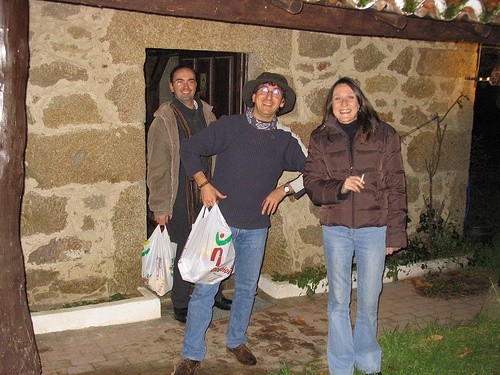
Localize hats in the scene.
[242,72,296,117]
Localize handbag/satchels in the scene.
[141,223,178,296]
[177,201,236,285]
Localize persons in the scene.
[172,72,308,375]
[145,63,234,323]
[304,76,406,375]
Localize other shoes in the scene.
[213,298,233,310]
[174,358,202,375]
[226,342,257,366]
[173,308,187,323]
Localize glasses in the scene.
[172,80,196,86]
[257,87,283,98]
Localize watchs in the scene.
[283,184,290,196]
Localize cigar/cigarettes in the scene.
[360,174,364,183]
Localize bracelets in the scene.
[199,180,209,189]
